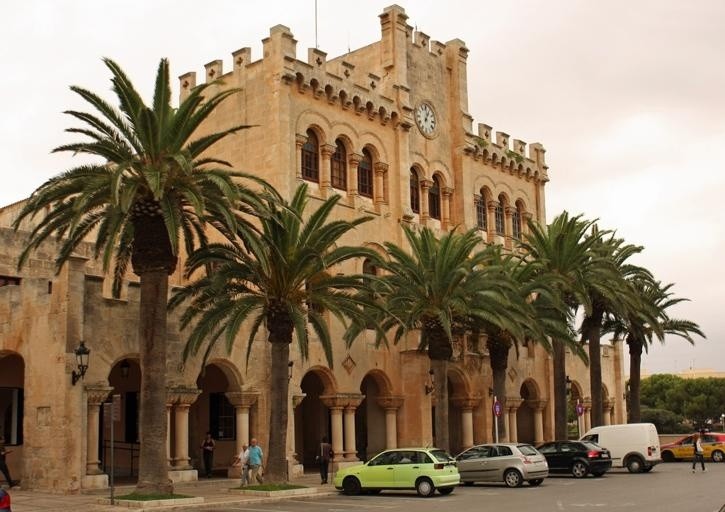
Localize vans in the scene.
[578,423,662,473]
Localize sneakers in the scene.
[691,467,708,473]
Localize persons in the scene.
[0,434,20,488]
[232,443,249,487]
[199,430,216,477]
[0,486,12,511]
[242,438,264,484]
[315,434,334,483]
[692,432,710,473]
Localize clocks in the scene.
[414,99,439,140]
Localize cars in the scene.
[536,439,612,478]
[453,442,549,487]
[660,433,725,463]
[334,447,461,496]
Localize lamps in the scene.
[566,375,572,395]
[71,342,90,386]
[425,369,435,395]
[288,360,293,382]
